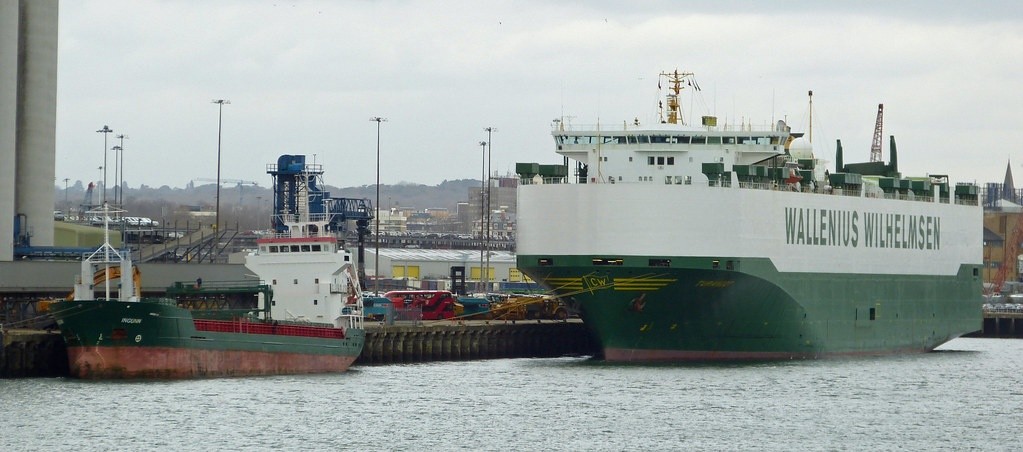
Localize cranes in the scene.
[984,207,1023,298]
[869,103,885,163]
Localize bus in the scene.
[382,289,456,320]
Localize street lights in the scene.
[110,145,123,219]
[95,125,113,212]
[478,140,489,296]
[483,123,500,290]
[369,115,389,297]
[62,178,72,221]
[116,134,130,221]
[211,98,231,268]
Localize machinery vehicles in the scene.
[35,265,142,317]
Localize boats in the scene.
[43,198,368,378]
[514,69,985,362]
